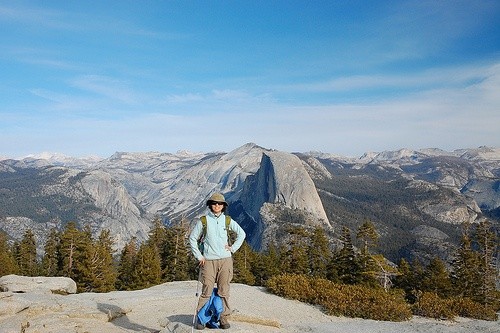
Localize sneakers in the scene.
[197,321,205,330]
[220,316,230,329]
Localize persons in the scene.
[189,192,246,330]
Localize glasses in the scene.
[211,201,223,205]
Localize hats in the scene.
[206,193,229,206]
